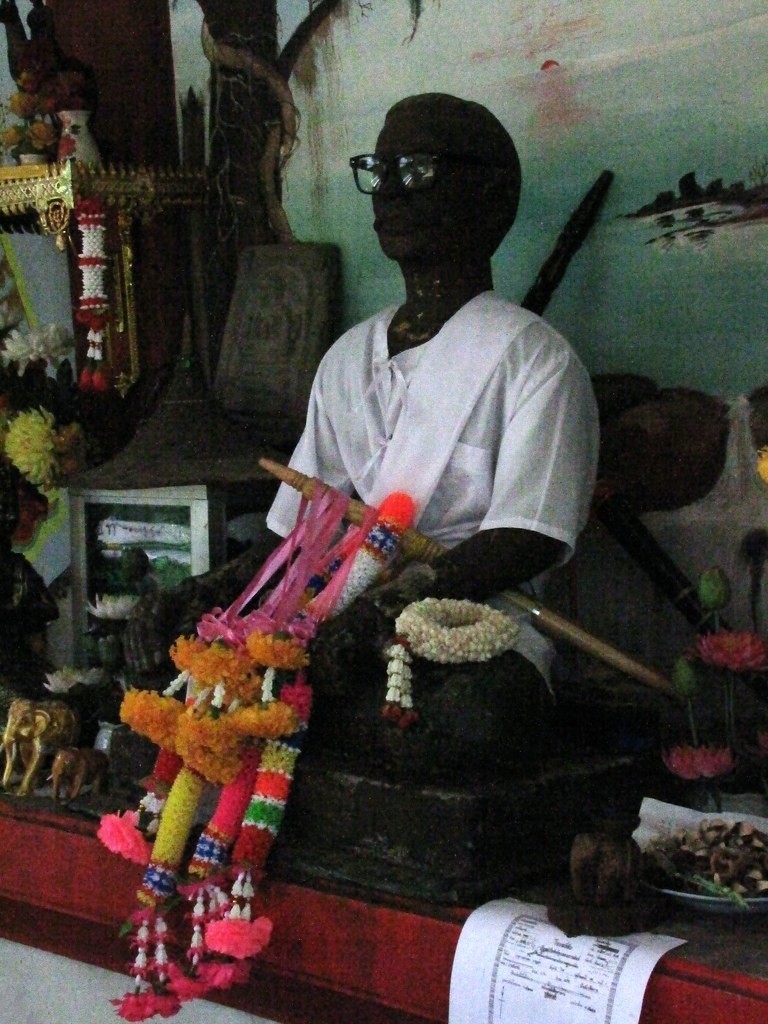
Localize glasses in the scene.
[350,148,504,194]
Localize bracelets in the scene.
[377,598,523,729]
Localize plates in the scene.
[643,881,767,913]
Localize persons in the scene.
[117,92,602,685]
